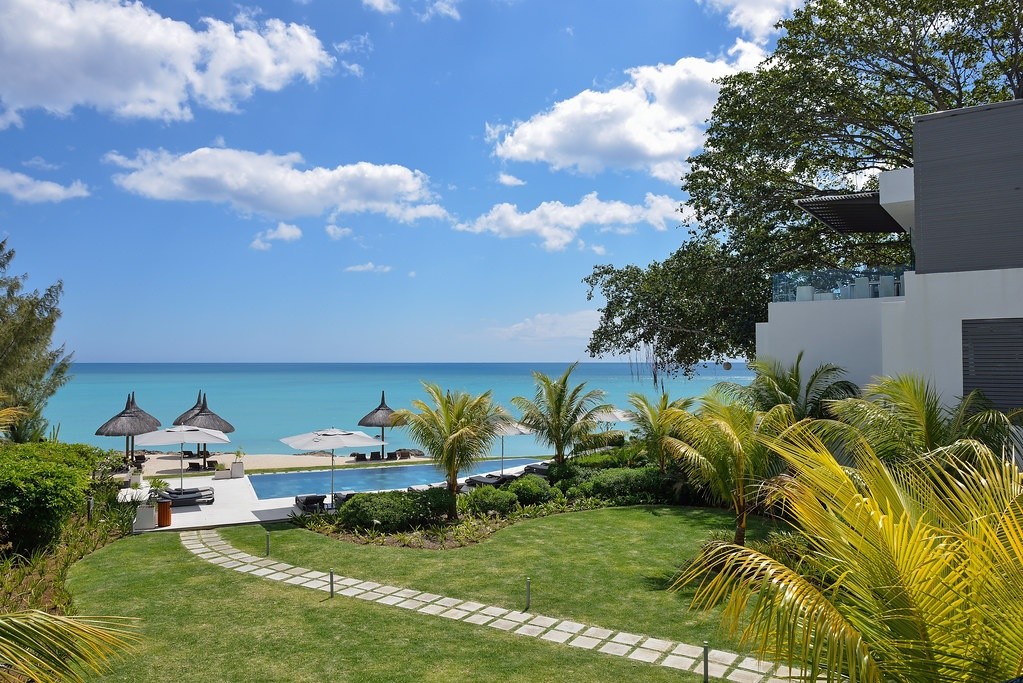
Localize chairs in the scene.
[132,462,142,471]
[186,462,204,471]
[370,451,382,461]
[180,450,216,458]
[295,494,328,515]
[524,462,549,474]
[386,451,411,460]
[334,493,362,506]
[134,455,150,463]
[408,482,463,494]
[464,472,535,488]
[356,452,367,461]
[149,487,215,507]
[207,460,224,471]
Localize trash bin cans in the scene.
[135,505,157,530]
[158,499,172,527]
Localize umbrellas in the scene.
[596,408,632,429]
[93,389,234,472]
[135,423,231,496]
[358,390,408,459]
[279,425,390,509]
[494,420,538,476]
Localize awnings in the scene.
[793,192,906,236]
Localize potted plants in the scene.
[147,475,170,504]
[213,462,232,478]
[230,447,247,478]
[129,469,143,489]
[136,496,157,529]
